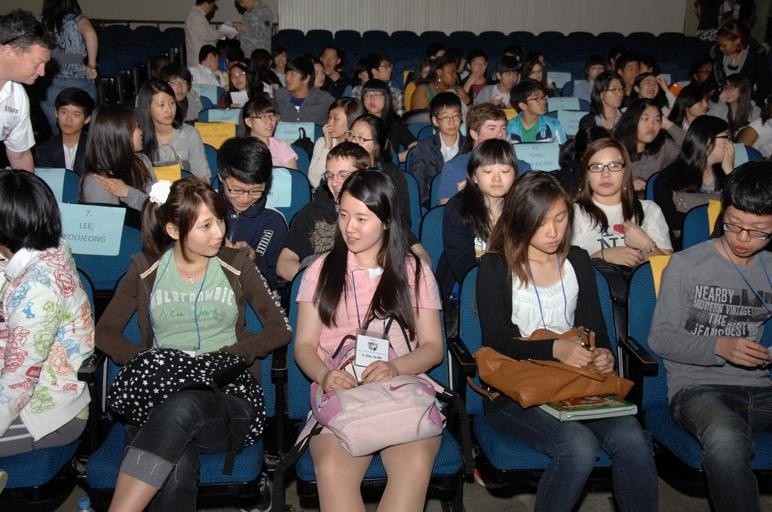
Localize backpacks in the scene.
[311,335,446,456]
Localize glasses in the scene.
[321,171,351,180]
[2,21,46,46]
[715,135,732,140]
[722,221,771,240]
[224,178,265,195]
[251,115,277,121]
[587,162,626,172]
[604,88,625,94]
[344,131,375,144]
[363,94,385,100]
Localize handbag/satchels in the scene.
[291,127,315,160]
[105,347,267,449]
[474,329,634,408]
[673,191,722,213]
[591,258,634,336]
[151,144,191,182]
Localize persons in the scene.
[35,45,771,487]
[92,178,290,512]
[2,1,97,173]
[476,171,657,511]
[184,1,273,68]
[648,157,771,511]
[293,170,446,512]
[1,169,94,496]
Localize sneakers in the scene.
[474,464,505,488]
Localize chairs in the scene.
[0,25,772,512]
